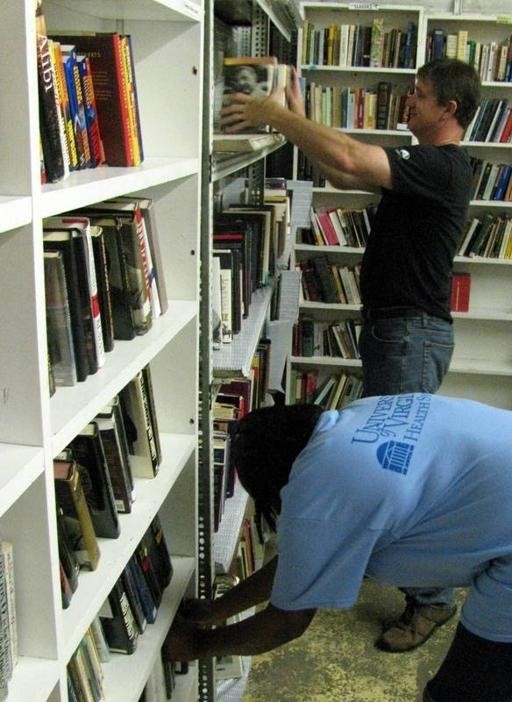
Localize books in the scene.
[0,666,9,700]
[0,553,13,680]
[2,539,20,667]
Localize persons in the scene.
[162,390,510,698]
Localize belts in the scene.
[359,306,436,321]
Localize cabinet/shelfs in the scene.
[420,13,512,413]
[283,1,428,405]
[0,0,209,702]
[206,1,307,702]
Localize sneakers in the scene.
[372,594,459,654]
